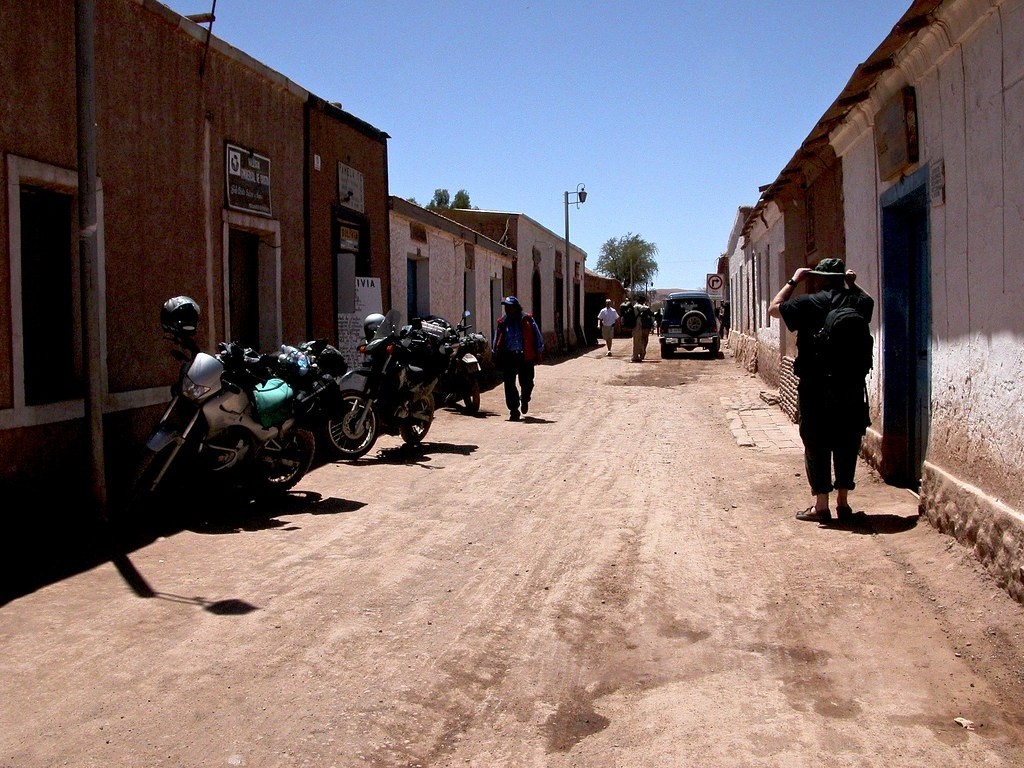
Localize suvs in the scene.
[657,292,721,359]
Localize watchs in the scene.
[786,278,797,288]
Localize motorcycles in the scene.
[323,307,461,460]
[113,293,347,522]
[438,308,489,414]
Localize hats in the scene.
[501,296,519,307]
[807,258,845,276]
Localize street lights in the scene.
[564,183,588,354]
[645,281,654,299]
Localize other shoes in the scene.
[641,355,644,359]
[795,505,832,522]
[606,351,611,356]
[632,358,641,363]
[509,410,520,420]
[836,504,853,520]
[521,398,528,414]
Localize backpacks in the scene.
[807,290,873,379]
[619,301,643,329]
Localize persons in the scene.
[654,309,663,337]
[768,258,875,522]
[631,296,655,361]
[719,296,730,340]
[597,299,621,356]
[491,295,543,420]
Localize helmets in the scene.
[363,313,394,344]
[160,295,201,337]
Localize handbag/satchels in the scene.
[298,340,348,378]
[449,331,487,371]
[294,376,341,431]
[218,340,272,381]
[400,314,454,366]
